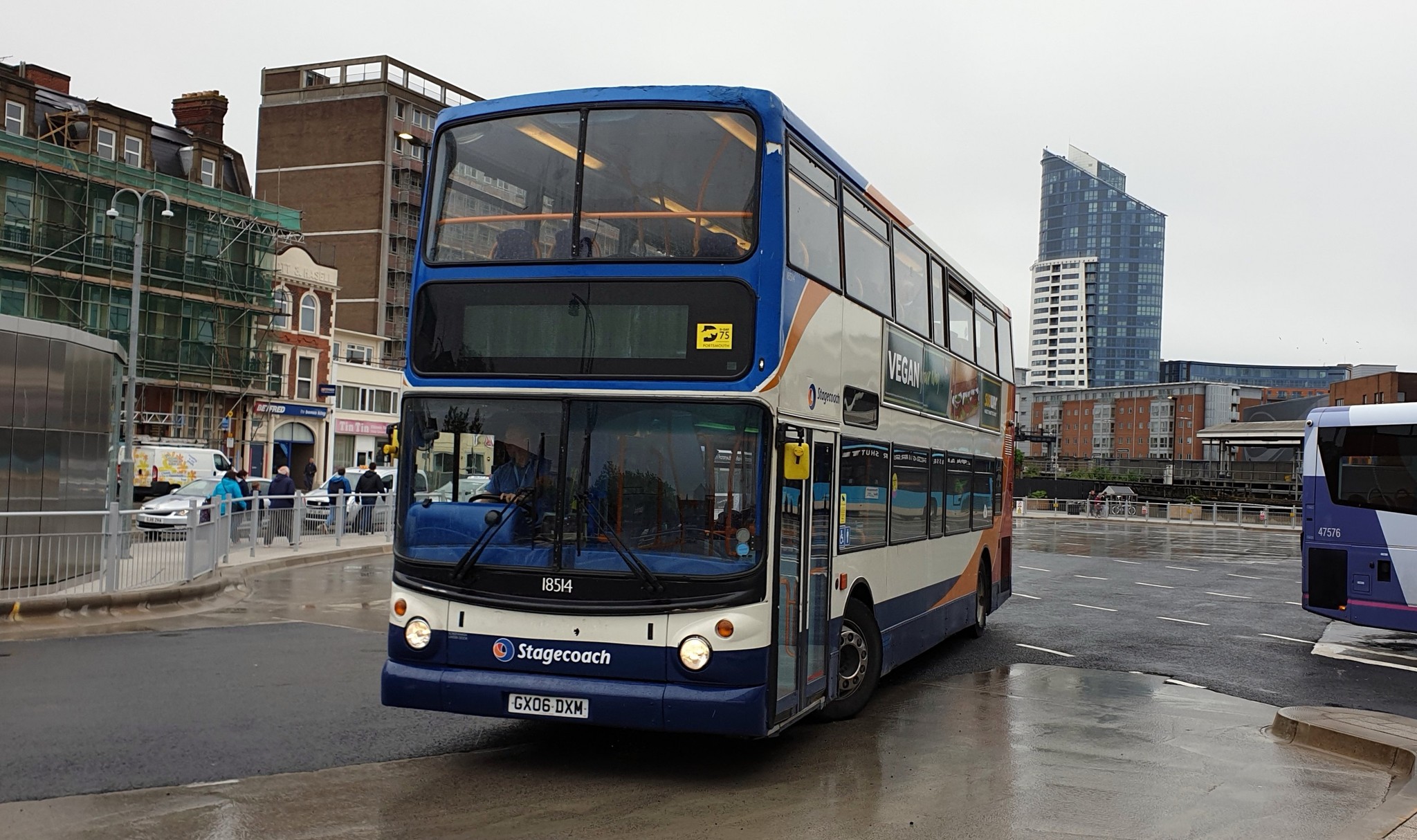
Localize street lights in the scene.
[1166,396,1179,485]
[106,187,175,562]
[567,292,598,374]
[1048,428,1058,512]
[1102,419,1115,459]
[1177,417,1192,476]
[1014,410,1018,480]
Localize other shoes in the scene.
[321,524,328,533]
[289,541,302,545]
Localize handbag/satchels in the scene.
[231,502,238,513]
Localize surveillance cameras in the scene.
[106,210,119,220]
[161,210,174,219]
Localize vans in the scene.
[106,434,242,502]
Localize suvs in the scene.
[303,466,429,532]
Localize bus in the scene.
[780,443,992,522]
[382,80,1018,742]
[1300,402,1417,635]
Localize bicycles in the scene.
[1111,495,1136,515]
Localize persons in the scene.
[302,458,316,492]
[1088,490,1107,518]
[355,462,386,536]
[323,468,351,534]
[595,437,679,545]
[480,425,553,511]
[212,469,265,547]
[264,466,295,548]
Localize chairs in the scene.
[1350,488,1414,508]
[488,228,970,368]
[713,505,961,544]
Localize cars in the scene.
[134,474,309,540]
[430,476,492,502]
